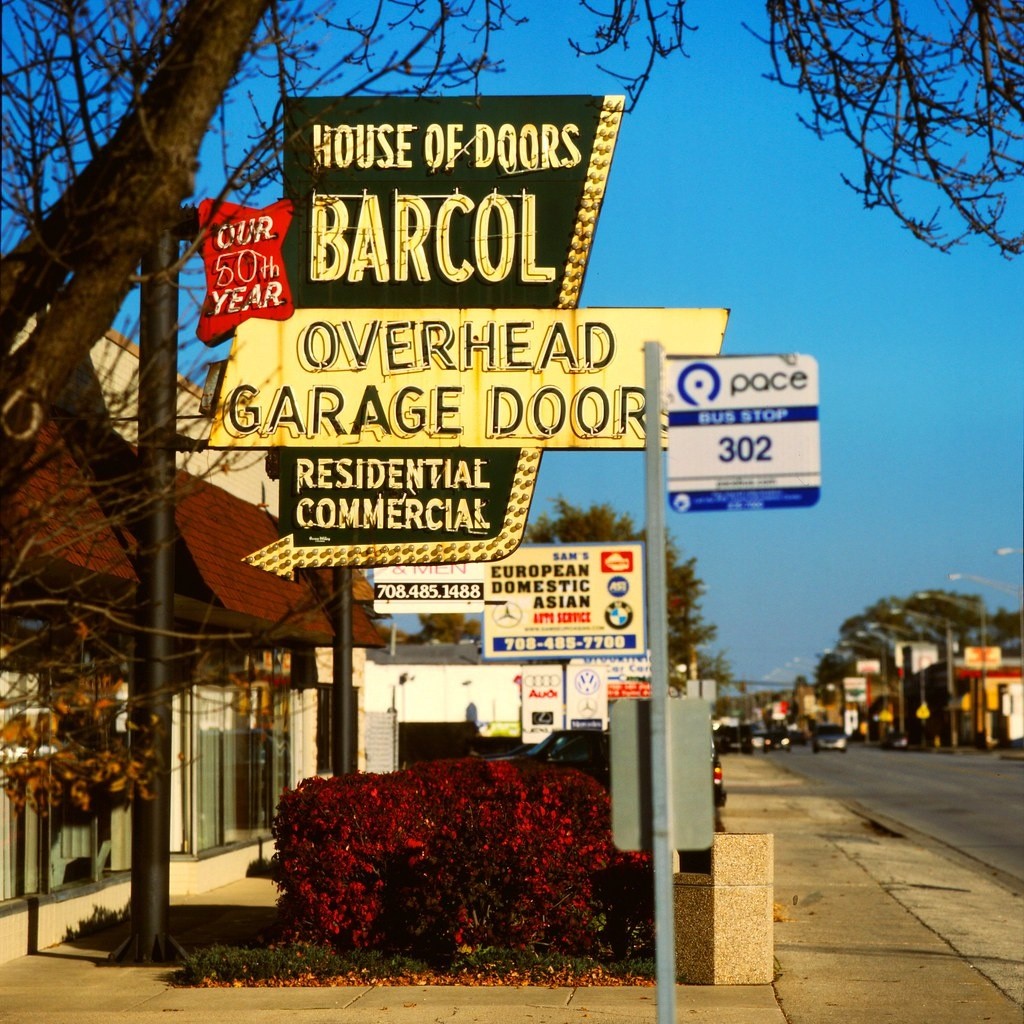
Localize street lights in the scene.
[948,574,1024,675]
[888,608,957,748]
[916,592,991,752]
[866,622,930,750]
[824,631,900,747]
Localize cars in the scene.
[811,724,848,754]
[713,719,806,754]
